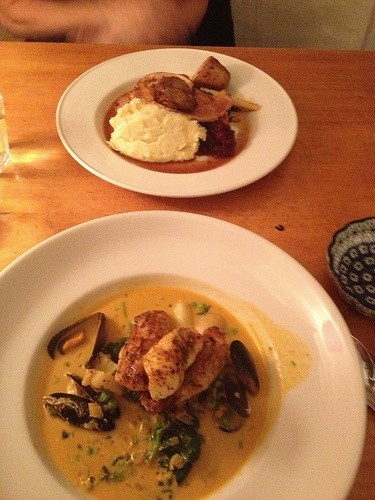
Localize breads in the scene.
[109,97,207,162]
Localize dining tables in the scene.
[0,41,375,500]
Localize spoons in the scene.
[352,335,375,411]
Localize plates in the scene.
[1,210,366,499]
[56,48,299,199]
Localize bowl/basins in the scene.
[328,217,375,317]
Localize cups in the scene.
[0,94,12,171]
